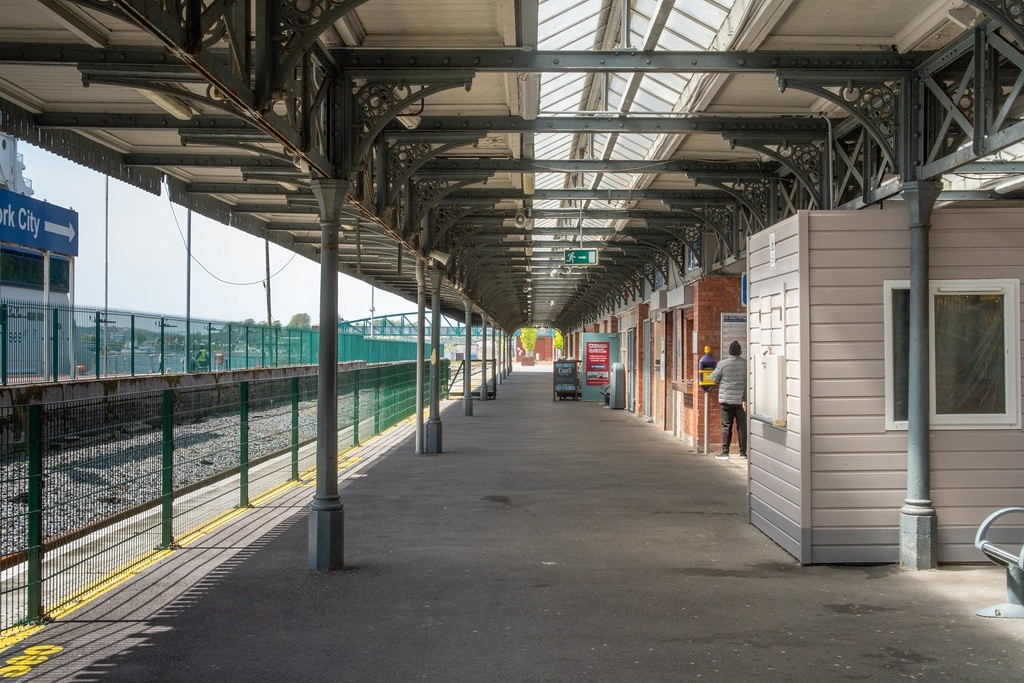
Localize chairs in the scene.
[974,507,1024,618]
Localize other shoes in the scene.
[737,453,747,459]
[715,453,729,460]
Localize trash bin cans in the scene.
[553,359,578,401]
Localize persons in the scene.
[158,351,166,372]
[214,349,224,371]
[181,353,185,372]
[195,342,209,372]
[708,340,747,460]
[255,358,262,366]
